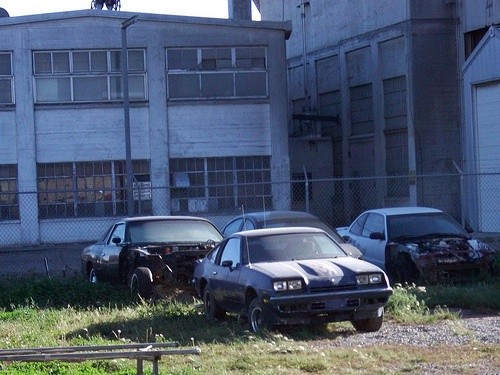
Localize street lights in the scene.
[119,15,138,218]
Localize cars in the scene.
[333,207,496,285]
[80,216,226,308]
[193,227,394,339]
[218,210,363,259]
